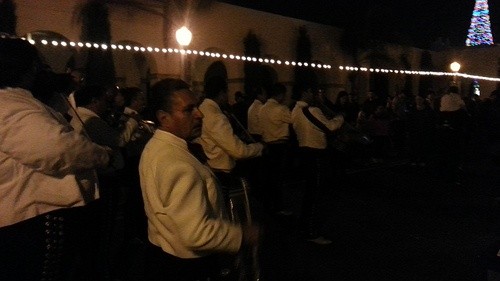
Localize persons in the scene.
[0,36,118,281]
[138,77,265,281]
[47,55,499,246]
